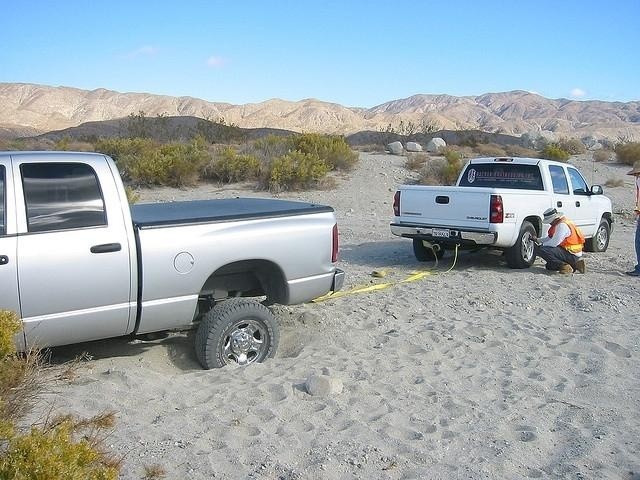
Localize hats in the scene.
[626,160,640,175]
[541,207,563,224]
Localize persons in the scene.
[625,159,640,276]
[529,207,586,275]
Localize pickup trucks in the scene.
[0,151,345,370]
[390,156,616,269]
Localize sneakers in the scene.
[558,262,574,275]
[574,258,586,274]
[626,268,640,276]
